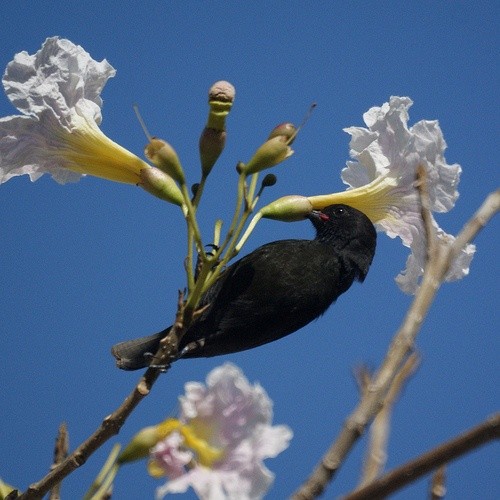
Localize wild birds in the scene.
[111,203,378,371]
